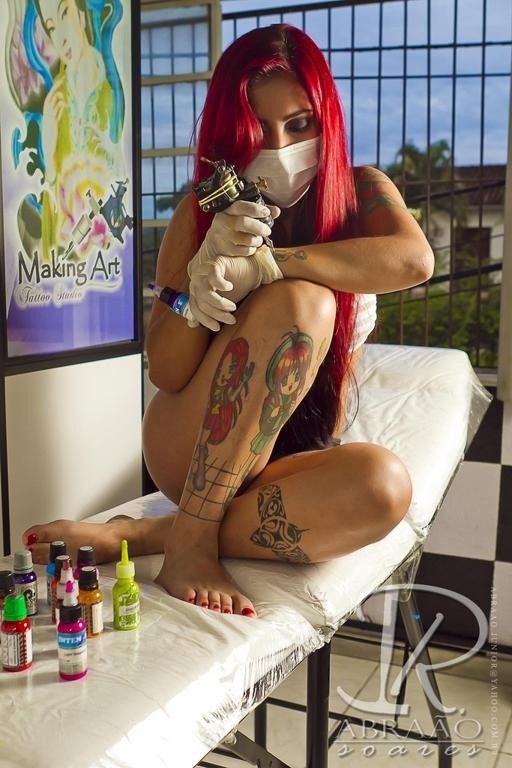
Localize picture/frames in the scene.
[0,0,145,378]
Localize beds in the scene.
[0,344,474,767]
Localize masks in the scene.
[241,134,320,209]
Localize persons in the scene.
[32,1,117,269]
[22,20,435,622]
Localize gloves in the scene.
[186,245,284,332]
[187,201,282,279]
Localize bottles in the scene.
[111,538,140,629]
[1,541,104,680]
[143,280,197,318]
